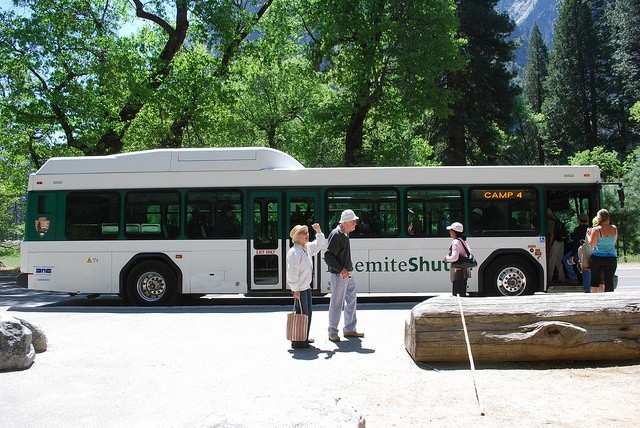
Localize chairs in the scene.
[162,224,170,238]
[126,223,141,234]
[200,224,208,238]
[140,223,162,235]
[101,222,119,235]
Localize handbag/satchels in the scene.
[448,253,477,268]
[286,313,308,341]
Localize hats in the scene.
[433,208,443,212]
[360,208,369,213]
[579,214,588,220]
[410,208,418,213]
[290,225,308,244]
[472,208,483,216]
[446,222,464,232]
[339,209,359,223]
[296,205,303,209]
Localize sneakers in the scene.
[306,339,314,343]
[566,279,578,283]
[344,332,364,337]
[292,341,309,348]
[329,334,340,341]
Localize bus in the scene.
[19,147,624,307]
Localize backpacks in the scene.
[547,218,569,242]
[576,231,593,273]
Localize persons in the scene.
[284,222,326,349]
[558,213,591,287]
[209,203,240,238]
[289,200,313,247]
[188,209,204,238]
[546,216,568,286]
[586,209,618,292]
[546,205,579,238]
[432,204,452,237]
[407,206,423,235]
[321,208,366,341]
[471,207,488,229]
[442,221,471,296]
[578,215,598,293]
